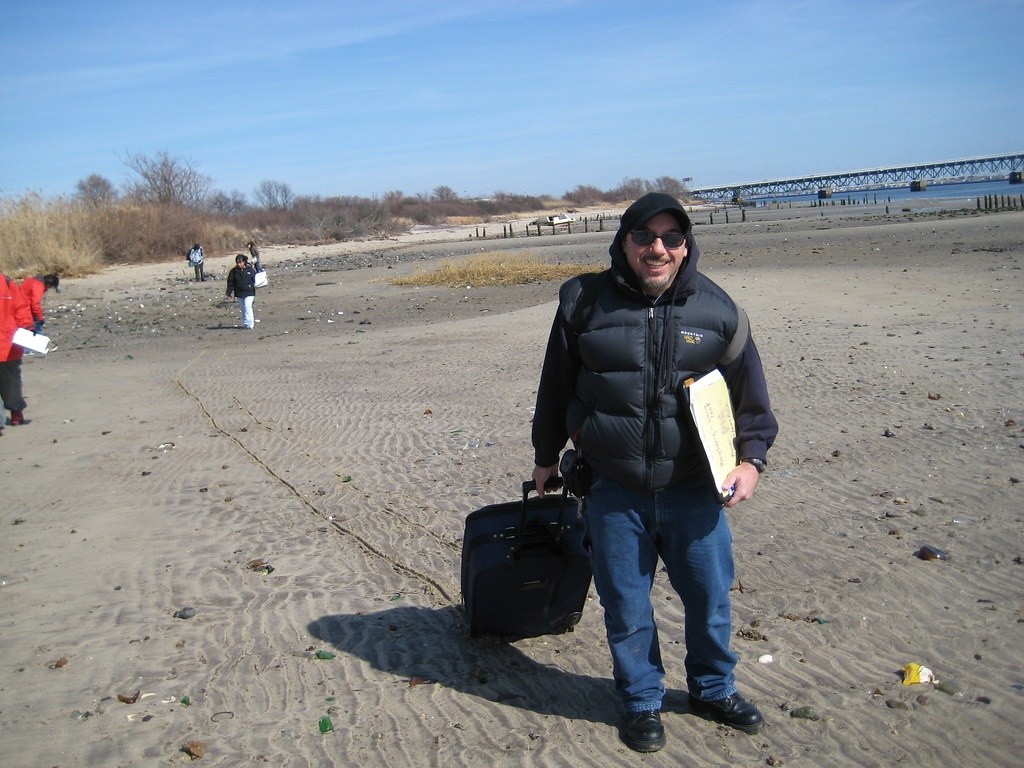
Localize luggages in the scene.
[458,478,592,647]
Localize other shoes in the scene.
[10,411,23,424]
[23,351,34,357]
[49,346,58,352]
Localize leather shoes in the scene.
[624,709,665,752]
[688,692,765,733]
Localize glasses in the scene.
[627,230,687,249]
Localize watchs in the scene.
[740,457,765,473]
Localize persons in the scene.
[246,241,264,273]
[0,271,38,426]
[531,192,780,753]
[189,243,207,282]
[0,395,5,433]
[19,272,62,356]
[226,254,268,329]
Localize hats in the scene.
[620,193,689,246]
